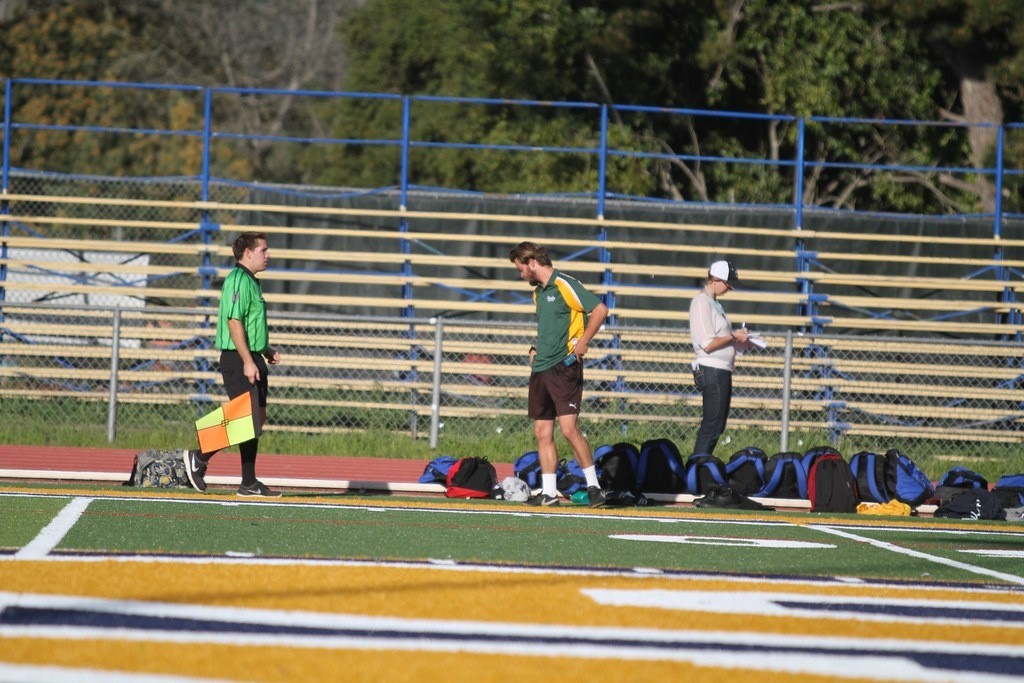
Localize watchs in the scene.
[528,345,537,353]
[730,334,738,343]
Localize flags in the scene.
[194,388,262,456]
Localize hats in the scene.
[710,261,743,292]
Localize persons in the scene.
[689,260,755,463]
[182,230,282,499]
[508,242,608,506]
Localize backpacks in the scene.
[445,456,497,499]
[418,457,459,484]
[514,438,1024,513]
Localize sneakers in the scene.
[522,491,560,507]
[587,486,607,508]
[237,480,282,497]
[182,449,207,493]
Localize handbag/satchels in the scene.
[934,492,1007,519]
[122,450,193,488]
[495,477,530,502]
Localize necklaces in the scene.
[703,284,725,314]
